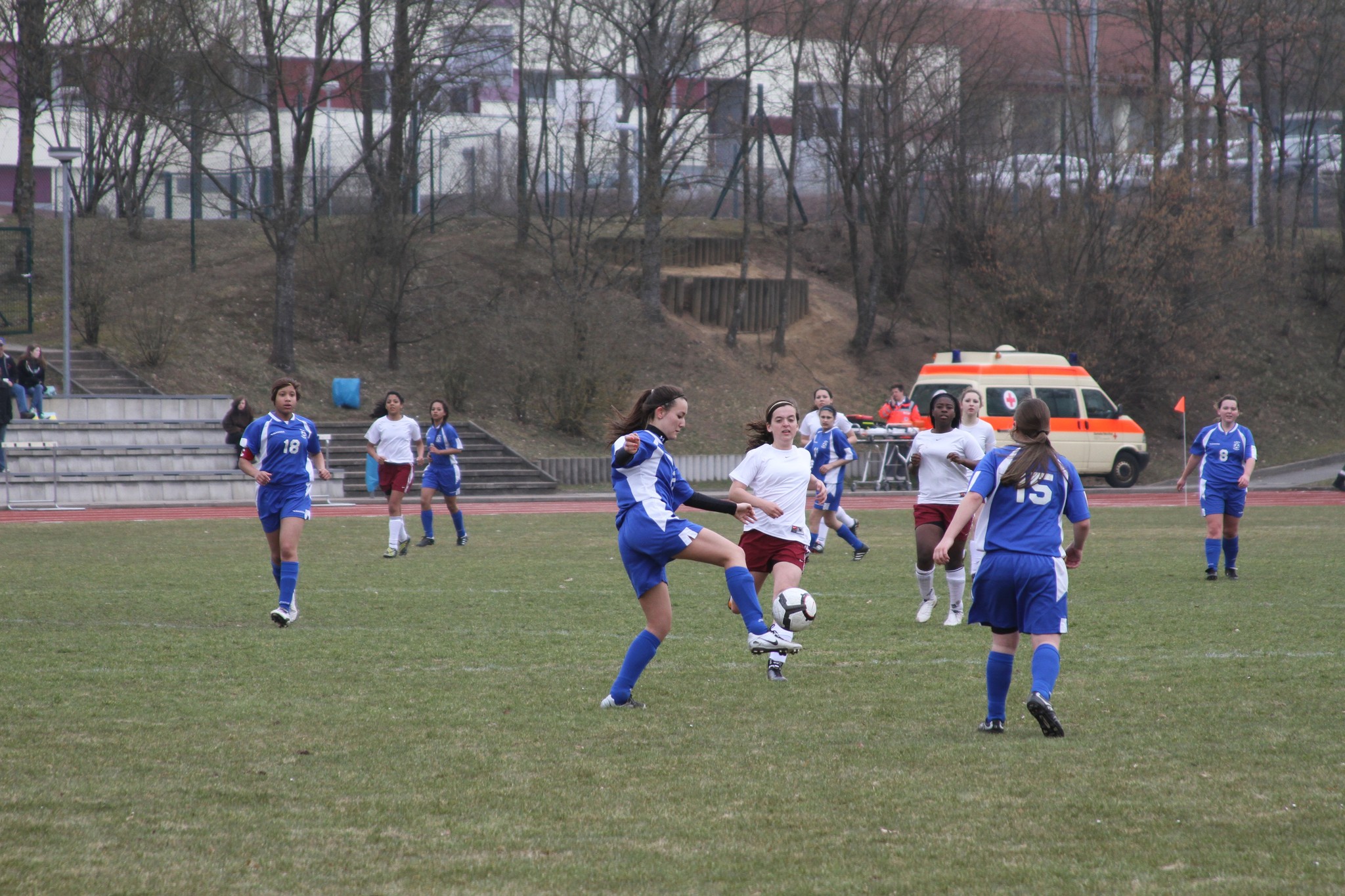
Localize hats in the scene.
[0,338,5,344]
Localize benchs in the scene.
[0,391,350,506]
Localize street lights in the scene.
[322,80,339,211]
[48,146,83,397]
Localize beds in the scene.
[849,423,921,488]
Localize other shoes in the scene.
[1,468,11,472]
[31,407,50,419]
[20,412,35,419]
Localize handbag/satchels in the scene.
[44,385,57,397]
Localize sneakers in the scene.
[416,536,435,547]
[851,543,870,562]
[747,630,803,656]
[1225,567,1239,579]
[917,595,937,623]
[399,538,410,556]
[456,532,468,546]
[811,541,824,553]
[270,609,290,629]
[766,668,789,684]
[383,547,398,558]
[944,610,964,626]
[1026,691,1065,739]
[288,589,298,623]
[848,518,860,540]
[1205,567,1218,580]
[978,717,1005,733]
[600,694,646,710]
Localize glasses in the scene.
[0,343,4,347]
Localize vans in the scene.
[909,344,1148,488]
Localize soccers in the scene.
[772,588,816,634]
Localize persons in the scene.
[934,396,1091,740]
[597,384,802,710]
[907,393,987,628]
[222,396,259,469]
[954,387,997,584]
[1175,394,1258,580]
[726,400,828,680]
[0,337,36,420]
[798,386,860,547]
[802,404,870,561]
[879,384,922,491]
[239,376,332,624]
[416,399,469,548]
[0,368,13,472]
[364,391,425,557]
[16,343,50,419]
